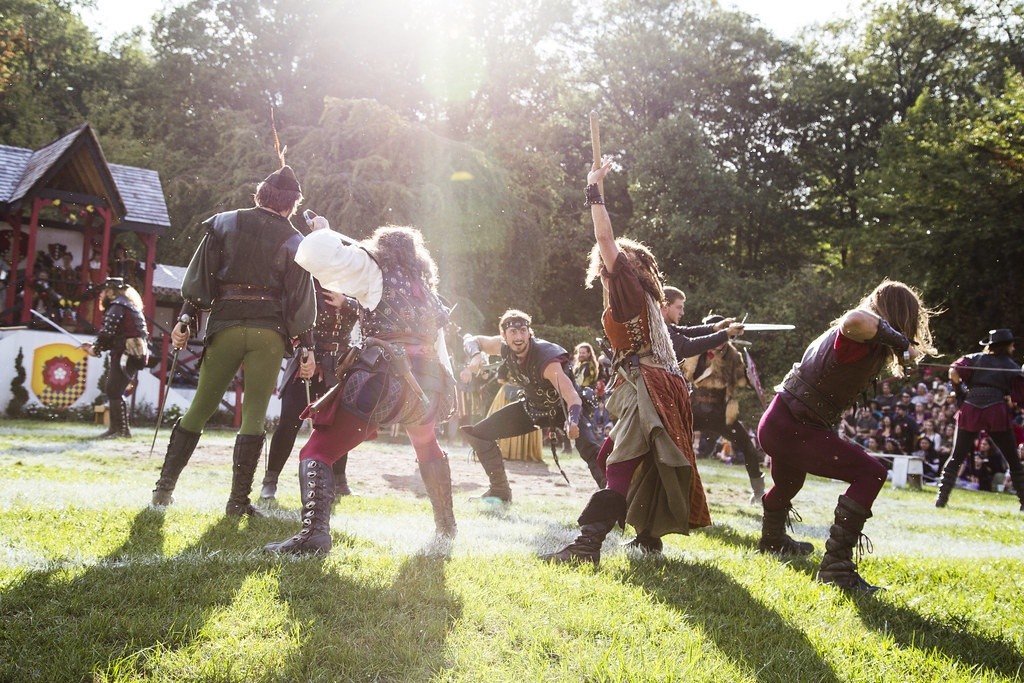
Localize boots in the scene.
[333,474,351,496]
[105,400,134,437]
[260,470,279,502]
[415,451,459,556]
[458,425,514,503]
[750,473,766,505]
[225,430,267,516]
[1009,469,1024,510]
[816,494,887,597]
[578,440,608,490]
[536,490,629,575]
[934,458,963,507]
[759,493,815,558]
[151,418,204,506]
[102,401,117,437]
[618,529,664,554]
[262,458,335,553]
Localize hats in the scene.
[96,277,129,290]
[263,165,302,193]
[979,329,1023,345]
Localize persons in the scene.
[443,286,768,505]
[839,362,1024,495]
[0,239,149,439]
[534,161,712,572]
[152,166,457,557]
[459,308,627,530]
[936,328,1024,511]
[755,278,937,594]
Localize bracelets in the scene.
[585,185,605,205]
